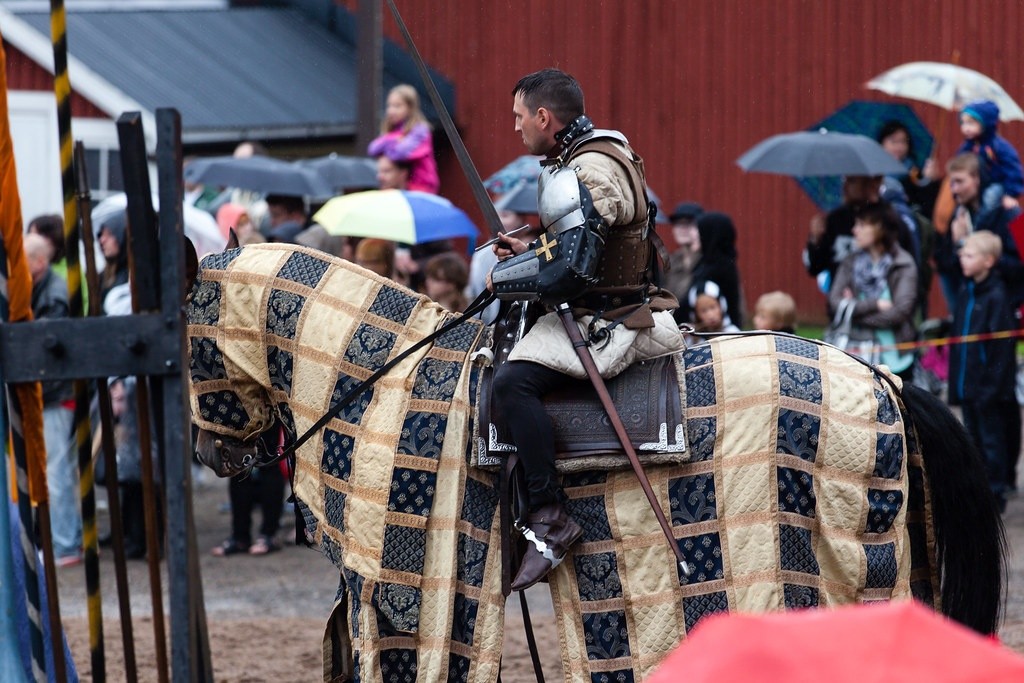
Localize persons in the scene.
[664,203,743,337]
[21,209,168,564]
[462,206,546,325]
[485,67,679,592]
[800,101,1024,517]
[178,142,469,556]
[368,84,440,193]
[752,290,797,334]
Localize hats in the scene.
[669,202,704,224]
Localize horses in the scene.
[184,224,1008,683]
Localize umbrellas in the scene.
[82,193,224,259]
[793,101,935,216]
[311,189,481,244]
[301,155,382,187]
[734,127,909,177]
[865,60,1024,124]
[181,155,325,196]
[482,155,671,223]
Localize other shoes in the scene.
[35,548,79,567]
[211,537,250,556]
[118,543,147,557]
[248,538,279,553]
[97,529,120,547]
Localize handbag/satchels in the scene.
[824,299,880,366]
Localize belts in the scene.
[569,284,649,311]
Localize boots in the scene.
[510,504,583,592]
